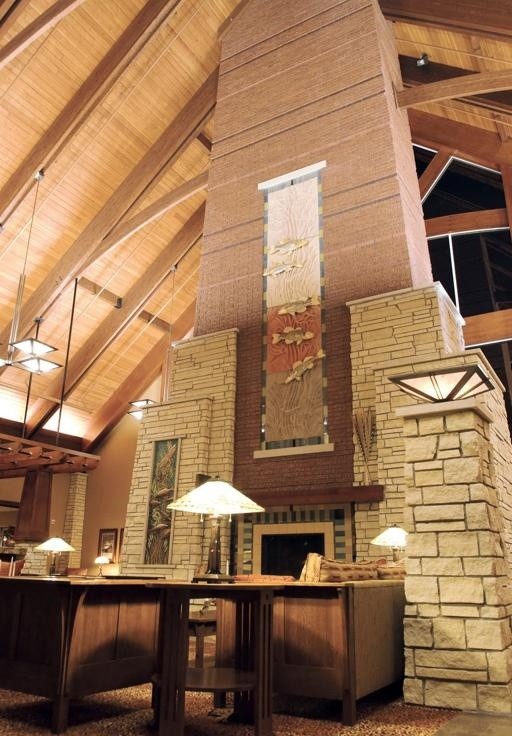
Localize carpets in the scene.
[0,639,462,736]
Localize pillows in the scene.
[298,552,405,583]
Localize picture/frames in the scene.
[97,527,126,564]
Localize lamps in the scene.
[386,363,494,403]
[165,478,265,585]
[6,315,64,375]
